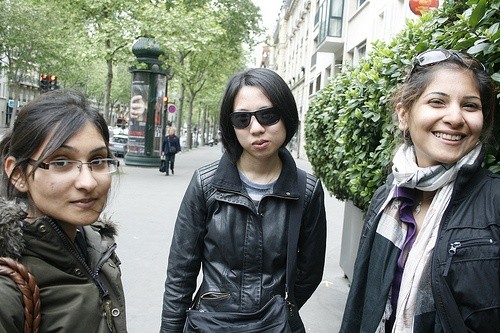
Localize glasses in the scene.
[28,150,119,177]
[228,105,281,129]
[411,48,486,73]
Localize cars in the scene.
[109,134,129,157]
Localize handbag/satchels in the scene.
[182,292,308,333]
[159,154,167,172]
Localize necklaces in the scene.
[414,197,435,215]
[239,160,280,185]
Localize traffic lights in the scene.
[40,74,49,94]
[49,75,57,91]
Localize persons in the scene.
[338,49,500,333]
[129,87,147,154]
[160,68,327,333]
[161,128,181,176]
[0,89,126,333]
